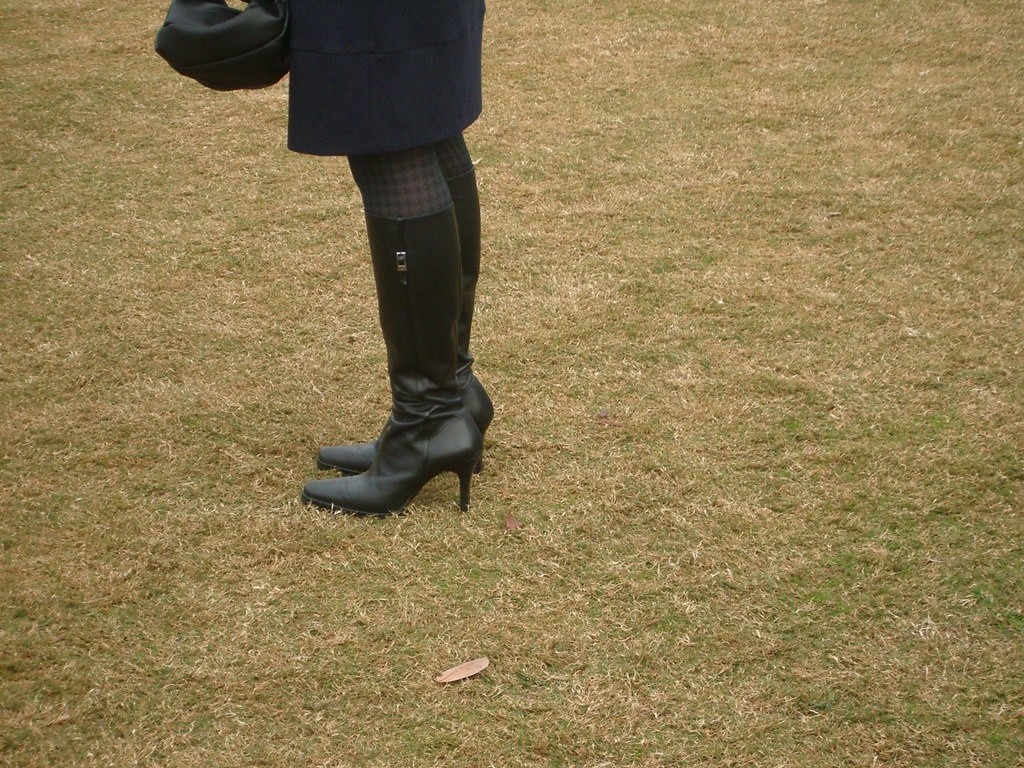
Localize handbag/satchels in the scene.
[155,0,290,91]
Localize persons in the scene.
[275,0,493,517]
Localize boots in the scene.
[299,160,496,515]
